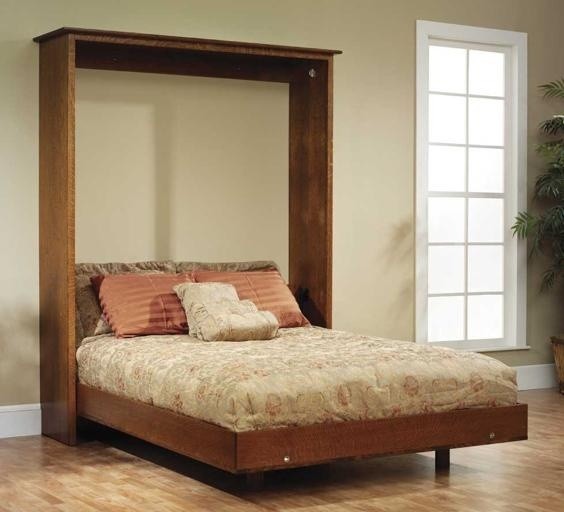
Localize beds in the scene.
[72,262,528,493]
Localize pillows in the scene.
[173,282,239,337]
[191,268,308,328]
[200,310,277,342]
[193,299,258,330]
[89,272,196,338]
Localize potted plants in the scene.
[510,78,564,393]
